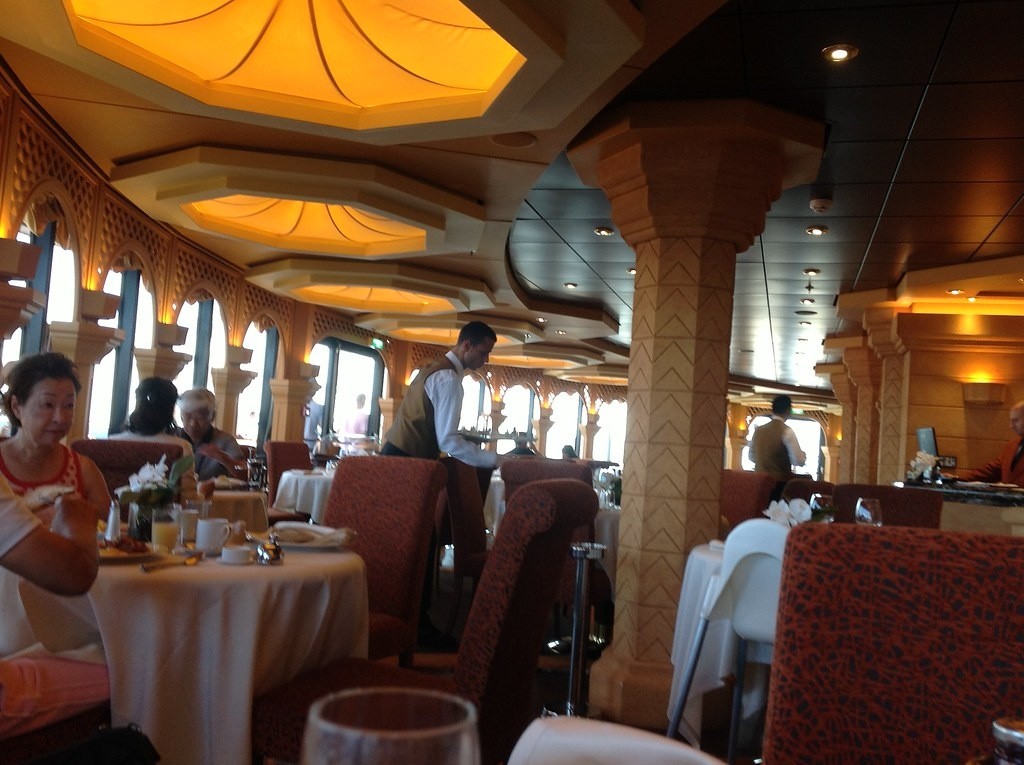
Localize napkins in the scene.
[708,538,726,553]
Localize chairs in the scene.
[1,438,1024,764]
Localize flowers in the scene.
[117,453,196,507]
[760,497,836,530]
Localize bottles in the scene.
[991,716,1024,765]
[613,469,622,506]
[104,499,122,548]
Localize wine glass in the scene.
[592,468,616,512]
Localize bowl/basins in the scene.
[222,546,250,562]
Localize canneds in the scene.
[990,715,1024,765]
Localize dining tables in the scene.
[273,469,336,524]
[664,540,778,765]
[590,504,621,599]
[482,477,507,535]
[81,526,367,765]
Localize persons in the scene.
[303,393,369,451]
[0,470,98,657]
[749,395,806,473]
[176,388,248,483]
[109,376,199,480]
[380,321,502,468]
[503,432,544,457]
[0,352,112,529]
[1008,401,1024,489]
[562,445,580,459]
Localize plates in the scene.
[97,520,341,564]
[215,478,244,488]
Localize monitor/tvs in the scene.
[918,428,939,484]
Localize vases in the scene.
[127,498,171,539]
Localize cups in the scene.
[296,686,483,765]
[185,499,213,519]
[195,518,231,551]
[809,493,834,523]
[854,497,882,526]
[151,503,183,553]
[182,509,199,541]
[328,459,341,474]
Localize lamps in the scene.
[0,0,1024,410]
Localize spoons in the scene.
[140,551,205,571]
